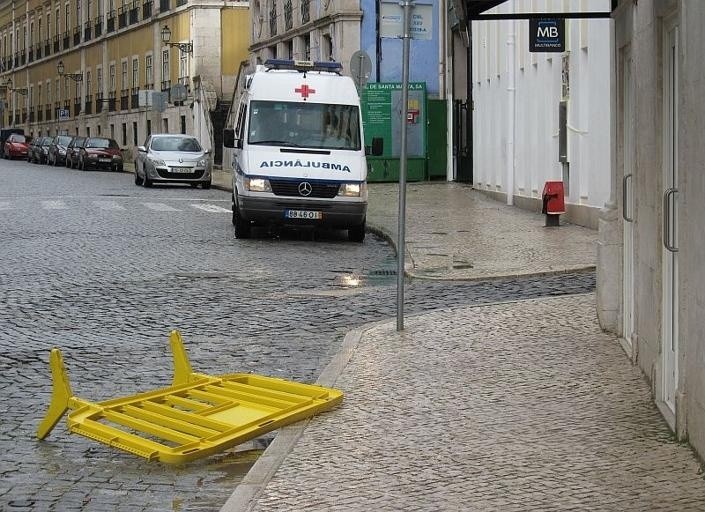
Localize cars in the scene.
[133,130,217,192]
[2,131,125,173]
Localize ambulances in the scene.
[222,56,373,244]
